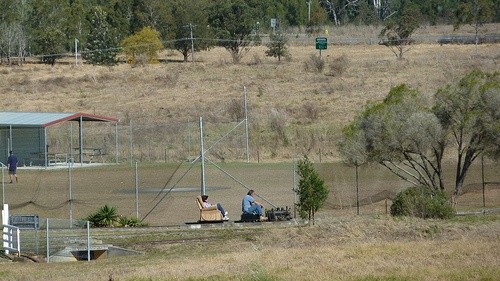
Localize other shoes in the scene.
[260,216,268,221]
[224,211,228,216]
[223,216,229,220]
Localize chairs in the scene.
[197,196,224,221]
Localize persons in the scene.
[242,190,268,221]
[7,150,18,183]
[202,195,230,220]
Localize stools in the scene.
[242,212,260,222]
